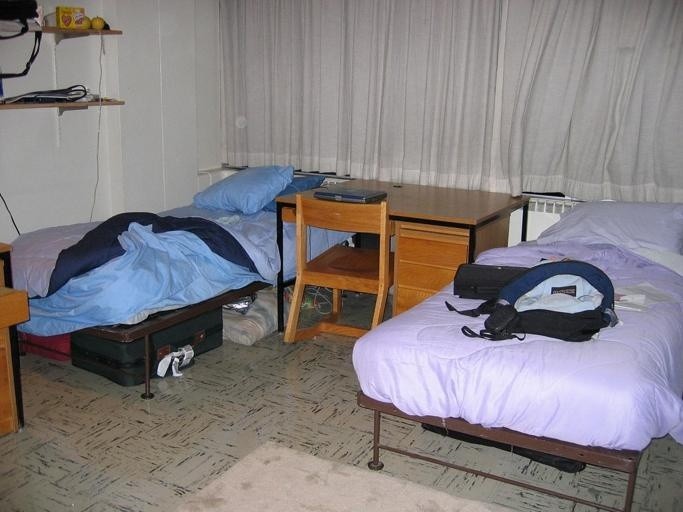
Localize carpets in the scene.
[173,442,513,511]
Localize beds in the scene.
[11,201,355,400]
[355,241,682,511]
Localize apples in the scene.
[82,16,104,29]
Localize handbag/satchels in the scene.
[453,259,533,301]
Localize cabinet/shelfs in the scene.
[0,20,125,108]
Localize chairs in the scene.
[284,192,395,347]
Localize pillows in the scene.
[192,164,325,217]
[536,200,683,254]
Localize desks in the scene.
[275,181,531,344]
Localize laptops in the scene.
[313,185,388,204]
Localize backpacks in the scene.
[474,257,616,343]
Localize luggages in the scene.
[15,296,224,389]
